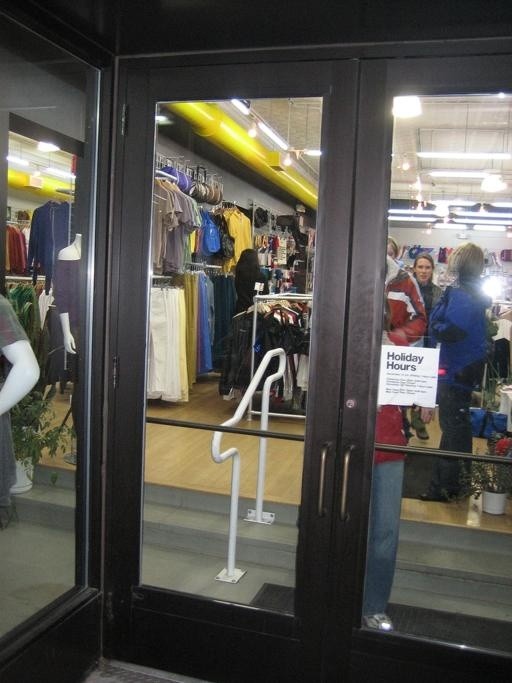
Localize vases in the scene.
[480,485,507,514]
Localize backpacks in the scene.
[195,206,221,257]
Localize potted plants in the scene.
[0,378,75,494]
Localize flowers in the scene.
[441,433,512,504]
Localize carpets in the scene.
[249,582,512,659]
[402,445,466,503]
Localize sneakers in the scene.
[365,613,393,630]
[420,486,455,501]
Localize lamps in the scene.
[388,92,512,239]
[230,99,321,169]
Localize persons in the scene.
[399,253,443,439]
[387,235,407,269]
[417,241,491,501]
[0,293,42,495]
[219,247,269,402]
[55,233,84,438]
[362,254,429,632]
[39,270,55,332]
[487,309,512,384]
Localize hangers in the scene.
[7,220,45,296]
[153,176,233,292]
[233,297,302,323]
